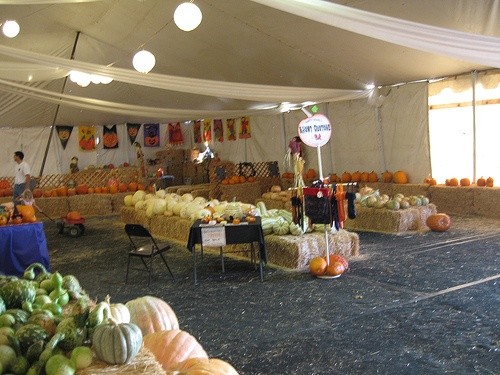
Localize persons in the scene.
[13,151,31,214]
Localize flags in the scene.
[193,120,202,144]
[226,119,237,141]
[144,124,161,147]
[239,116,252,138]
[78,126,96,152]
[103,124,119,149]
[168,122,183,145]
[214,120,224,143]
[203,120,212,141]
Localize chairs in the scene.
[125,224,176,287]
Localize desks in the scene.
[188,215,267,285]
[0,223,51,279]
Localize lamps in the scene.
[132,46,156,74]
[173,2,202,31]
[2,19,20,38]
[70,71,113,87]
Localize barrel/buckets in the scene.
[156,178,171,189]
